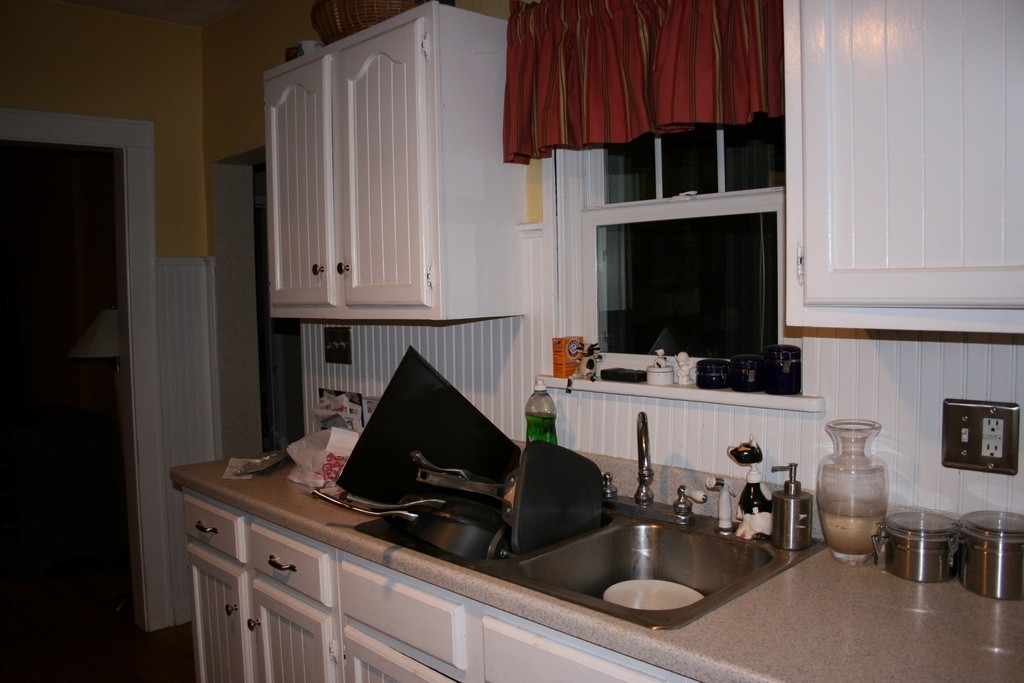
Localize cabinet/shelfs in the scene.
[337,548,667,683]
[783,0,1024,334]
[181,485,345,683]
[264,0,527,321]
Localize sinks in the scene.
[519,504,828,632]
[351,495,631,571]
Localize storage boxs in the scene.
[553,336,584,377]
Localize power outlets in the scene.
[940,399,1020,476]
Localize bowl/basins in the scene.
[693,345,802,396]
[957,509,1023,601]
[871,511,960,583]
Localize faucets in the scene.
[630,411,655,517]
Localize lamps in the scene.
[66,302,133,611]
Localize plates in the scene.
[603,579,704,610]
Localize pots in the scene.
[312,439,605,561]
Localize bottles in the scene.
[814,418,890,567]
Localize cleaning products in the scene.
[727,436,774,541]
[523,376,557,445]
[769,463,814,552]
